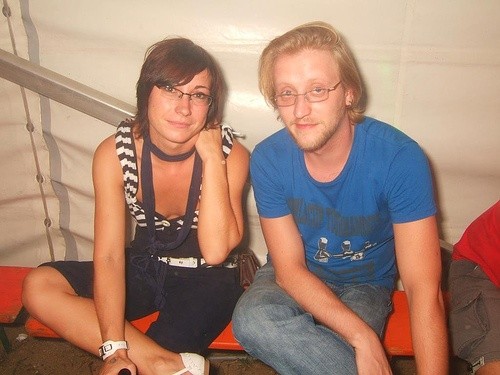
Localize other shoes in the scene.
[173,353,210,375]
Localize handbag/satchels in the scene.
[239,249,261,289]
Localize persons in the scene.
[231,20,449,375]
[21,38,256,375]
[452,201,500,375]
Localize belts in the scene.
[151,250,240,268]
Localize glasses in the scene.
[271,80,342,106]
[156,84,213,107]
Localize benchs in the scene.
[0,265,456,355]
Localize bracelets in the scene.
[99,339,127,360]
